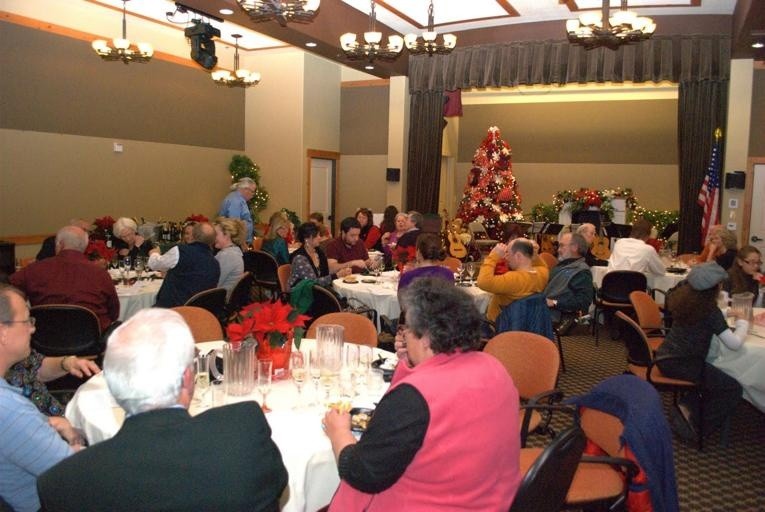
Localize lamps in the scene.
[220,9,234,16]
[365,65,374,71]
[753,42,762,50]
[405,32,458,58]
[237,0,320,27]
[306,42,316,48]
[566,12,631,49]
[211,68,263,88]
[611,11,657,46]
[113,38,153,64]
[340,31,404,63]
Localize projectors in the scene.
[183,24,221,70]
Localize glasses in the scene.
[5,316,38,326]
[743,259,763,266]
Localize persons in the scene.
[5,349,101,446]
[545,233,594,326]
[35,217,90,265]
[112,217,153,267]
[309,212,330,242]
[379,205,399,234]
[706,228,738,270]
[9,226,120,334]
[326,216,371,275]
[381,212,408,260]
[355,208,382,251]
[656,260,749,443]
[261,211,292,266]
[477,238,550,337]
[724,246,765,307]
[605,220,665,339]
[148,222,220,309]
[322,276,520,512]
[36,309,289,512]
[219,177,256,247]
[395,211,423,249]
[0,285,85,512]
[577,223,608,267]
[215,219,246,303]
[397,232,455,311]
[181,222,197,244]
[286,222,351,290]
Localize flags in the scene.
[697,128,722,246]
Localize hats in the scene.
[686,260,729,291]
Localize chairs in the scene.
[243,247,282,302]
[278,258,298,299]
[630,286,681,333]
[482,329,555,448]
[539,251,559,276]
[305,311,379,349]
[542,308,582,374]
[228,271,254,315]
[301,286,381,346]
[164,305,224,343]
[28,304,103,393]
[592,268,658,348]
[182,287,227,323]
[503,375,671,512]
[611,311,733,451]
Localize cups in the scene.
[467,263,475,283]
[124,257,131,280]
[374,265,379,285]
[316,325,344,372]
[257,359,272,414]
[361,344,374,370]
[346,344,359,370]
[134,259,143,281]
[309,351,321,393]
[457,263,465,283]
[119,260,125,276]
[208,341,257,396]
[291,352,305,396]
[197,357,209,390]
[112,259,118,268]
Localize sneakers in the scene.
[673,400,699,442]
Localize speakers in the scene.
[386,167,401,182]
[725,171,746,191]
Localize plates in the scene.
[343,279,359,284]
[362,280,376,283]
[371,358,395,373]
[348,407,374,432]
